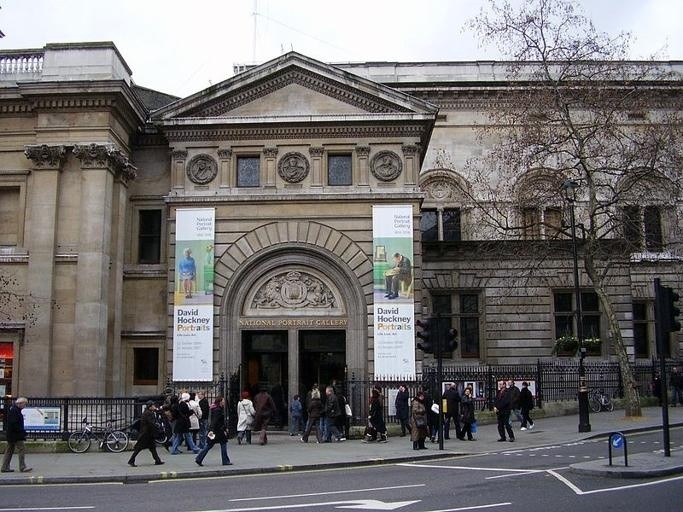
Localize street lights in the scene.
[561,178,594,433]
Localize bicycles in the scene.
[66,414,129,454]
[586,387,614,413]
[152,409,172,445]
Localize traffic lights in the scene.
[438,320,459,354]
[661,284,681,332]
[415,318,436,354]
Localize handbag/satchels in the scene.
[132,417,144,433]
[245,415,259,426]
[345,404,352,418]
[430,403,440,414]
[415,416,427,430]
[471,422,477,433]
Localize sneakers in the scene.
[155,461,166,465]
[20,467,33,472]
[127,461,137,467]
[222,462,234,465]
[497,438,506,442]
[529,422,534,429]
[509,437,515,442]
[162,440,171,453]
[520,426,528,431]
[237,431,478,451]
[171,446,203,456]
[192,458,204,467]
[1,468,15,472]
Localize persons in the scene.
[289,378,350,442]
[381,252,412,299]
[194,396,232,466]
[493,377,535,442]
[126,378,209,467]
[0,395,32,473]
[360,384,387,443]
[395,377,477,449]
[667,365,683,407]
[236,378,284,445]
[177,247,197,299]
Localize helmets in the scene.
[241,392,249,398]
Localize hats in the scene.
[145,400,156,409]
